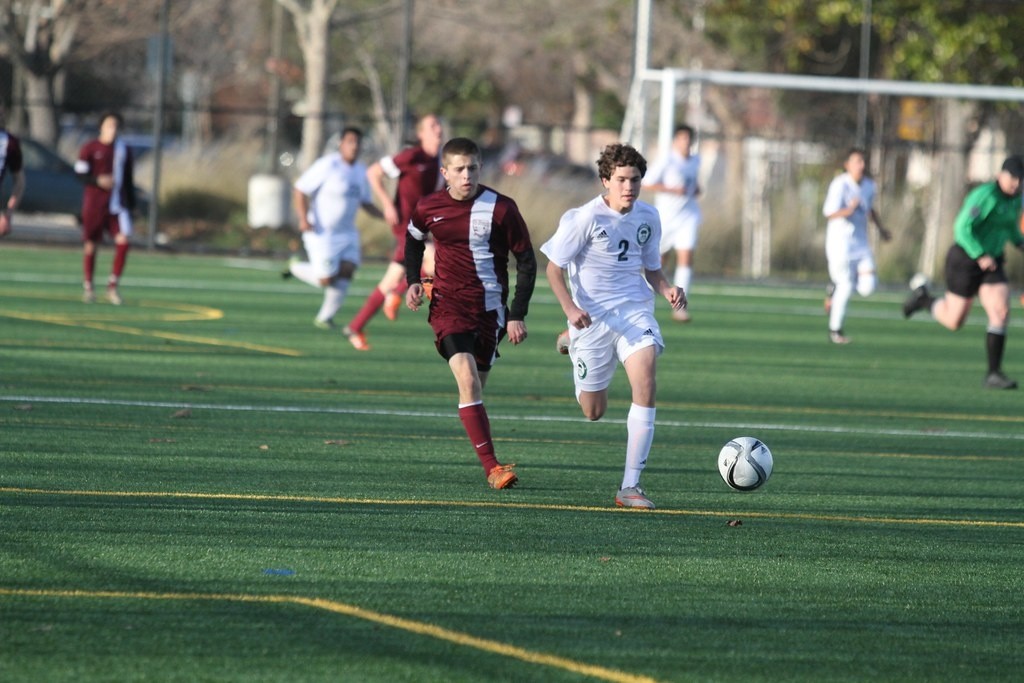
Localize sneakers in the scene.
[557,329,570,354]
[615,483,656,508]
[488,464,518,490]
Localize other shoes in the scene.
[383,291,402,320]
[984,371,1018,389]
[420,275,434,301]
[901,286,927,319]
[311,316,336,331]
[281,255,301,278]
[344,326,370,350]
[825,296,831,315]
[673,306,690,321]
[105,290,121,305]
[828,333,850,345]
[84,289,96,304]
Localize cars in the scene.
[0,137,159,230]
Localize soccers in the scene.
[716,436,774,492]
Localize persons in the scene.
[539,143,688,510]
[0,103,25,237]
[281,126,386,331]
[640,125,703,321]
[344,114,445,348]
[74,110,137,303]
[905,157,1024,387]
[823,149,891,344]
[404,138,536,490]
